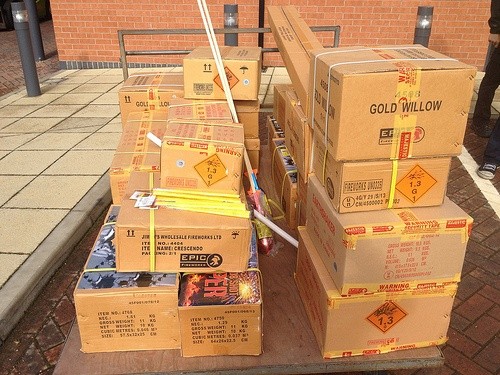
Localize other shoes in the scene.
[470,122,493,137]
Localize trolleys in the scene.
[51,24,442,375]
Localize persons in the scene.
[471,0,500,137]
[477,115,500,180]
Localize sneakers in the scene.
[477,161,497,180]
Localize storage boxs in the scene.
[267,4,477,359]
[74,46,262,358]
